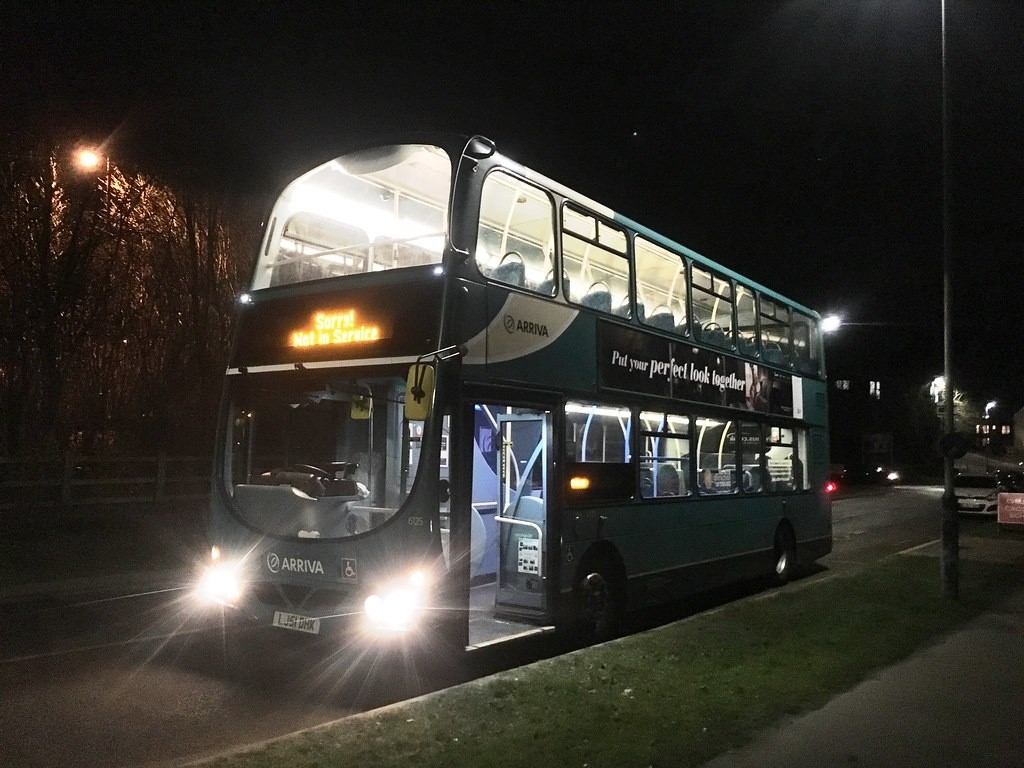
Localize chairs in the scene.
[702,322,725,348]
[746,336,768,361]
[745,466,772,491]
[490,251,526,286]
[536,267,570,300]
[615,294,645,323]
[646,304,674,332]
[766,342,784,365]
[657,464,679,496]
[580,281,612,313]
[725,329,745,354]
[675,314,703,341]
[784,351,800,371]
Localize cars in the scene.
[940,467,1011,521]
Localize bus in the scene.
[207,128,832,670]
